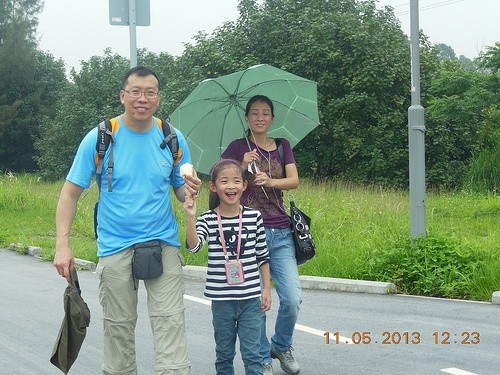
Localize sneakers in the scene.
[270,342,300,375]
[263,363,273,375]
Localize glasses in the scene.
[124,87,159,99]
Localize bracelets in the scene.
[194,191,200,199]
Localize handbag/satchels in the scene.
[134,240,163,279]
[289,200,315,265]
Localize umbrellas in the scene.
[168,64,321,174]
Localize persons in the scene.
[54,66,201,375]
[221,96,302,375]
[183,159,271,375]
